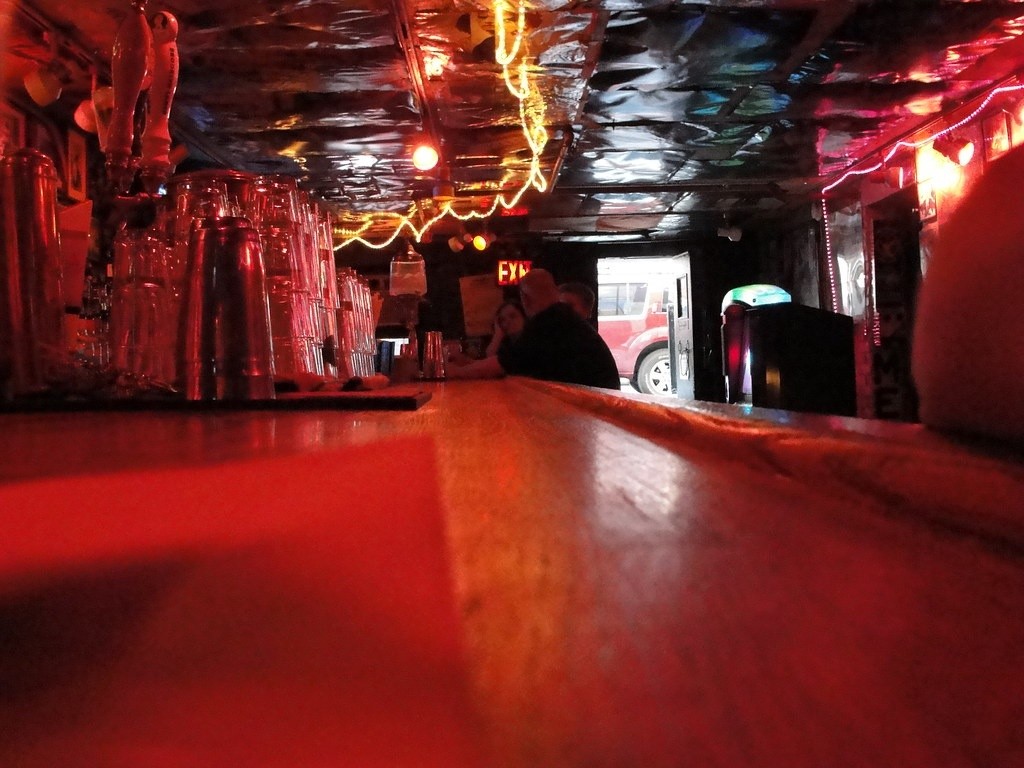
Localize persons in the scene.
[444,269,620,390]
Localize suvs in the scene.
[599,274,673,395]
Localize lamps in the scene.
[19,63,73,108]
[717,217,742,243]
[74,67,99,135]
[998,91,1024,124]
[931,125,977,165]
[472,219,498,250]
[447,223,474,254]
[867,153,905,191]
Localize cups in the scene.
[0,147,380,399]
[442,339,460,377]
